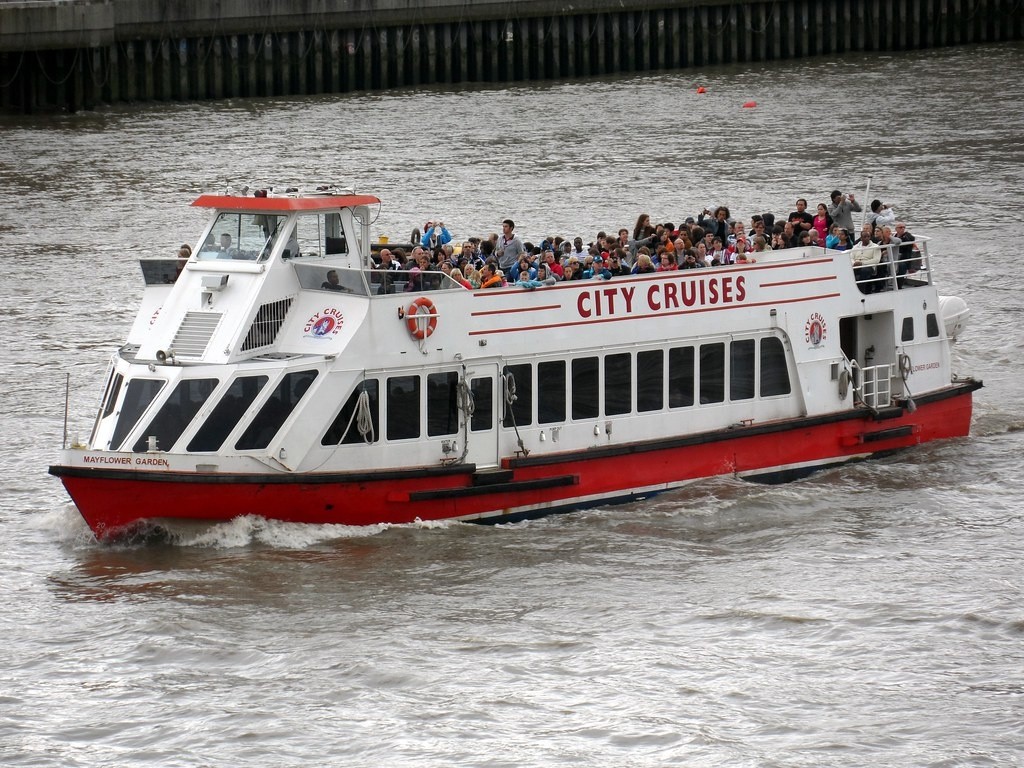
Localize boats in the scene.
[47,185,986,545]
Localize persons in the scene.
[272,225,299,258]
[220,233,237,256]
[321,270,355,293]
[367,190,916,296]
[124,378,460,453]
[201,234,221,252]
[178,248,190,258]
[180,243,192,256]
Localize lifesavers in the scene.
[405,297,437,338]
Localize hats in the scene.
[593,255,603,262]
